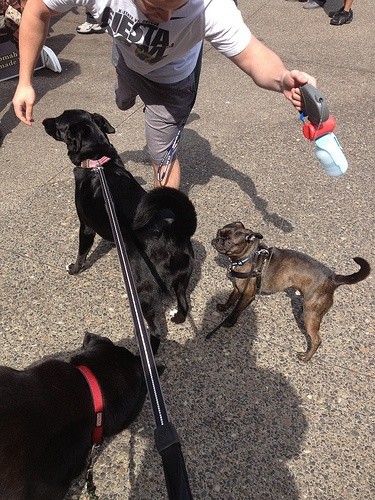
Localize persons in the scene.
[12,0,317,190]
[298,0,354,25]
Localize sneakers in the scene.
[76,21,102,33]
[330,9,353,25]
[303,0,320,9]
[329,6,351,18]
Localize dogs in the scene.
[42,109,198,354]
[210,222,371,362]
[0,332,165,500]
